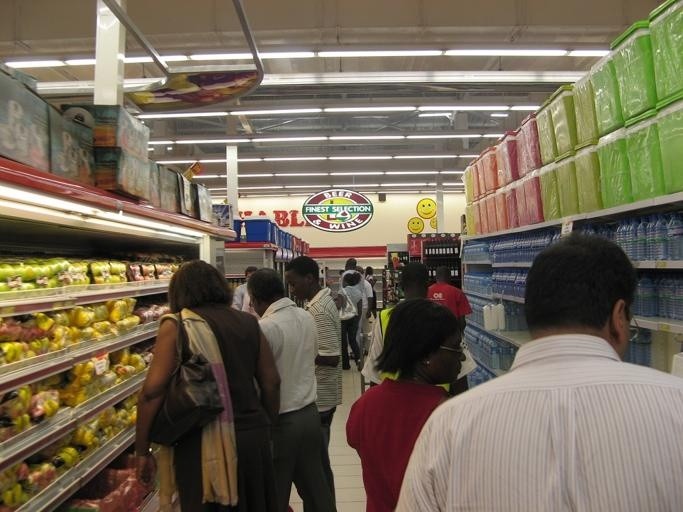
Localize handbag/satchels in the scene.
[338,287,358,321]
[148,310,226,447]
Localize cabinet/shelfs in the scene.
[225,242,278,306]
[0,155,238,512]
[462,193,682,411]
[420,238,462,284]
[319,268,383,310]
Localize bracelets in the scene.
[136,448,152,457]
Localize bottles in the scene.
[462,228,560,297]
[623,326,652,367]
[424,241,460,286]
[579,212,682,321]
[459,295,528,389]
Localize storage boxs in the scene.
[649,0,682,193]
[497,112,545,230]
[0,59,213,223]
[571,20,664,214]
[462,147,507,237]
[225,219,309,255]
[533,85,580,222]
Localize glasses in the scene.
[248,296,255,308]
[628,313,640,341]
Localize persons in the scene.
[246,267,337,512]
[426,266,472,336]
[135,261,281,512]
[346,296,466,512]
[285,256,343,512]
[320,258,377,371]
[361,262,451,393]
[230,266,260,320]
[395,229,683,512]
